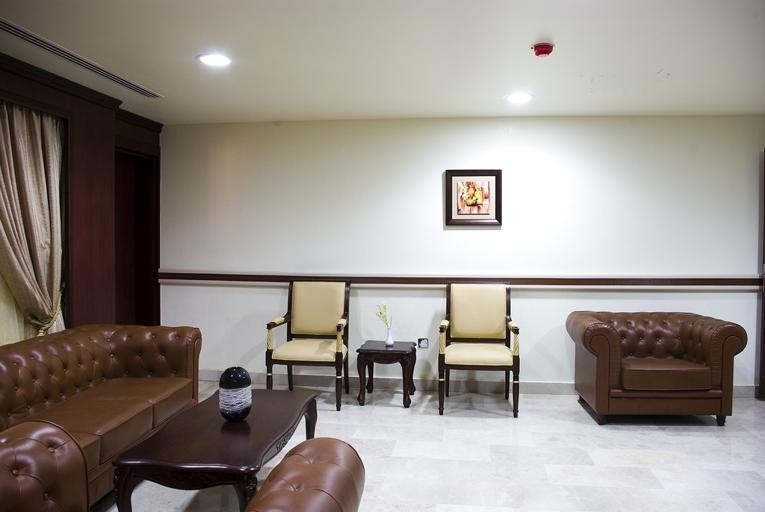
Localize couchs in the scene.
[0,324,202,512]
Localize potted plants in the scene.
[376,305,394,347]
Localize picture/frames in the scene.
[446,169,502,226]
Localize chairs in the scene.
[567,311,748,425]
[437,283,520,418]
[266,280,350,410]
[0,421,366,512]
